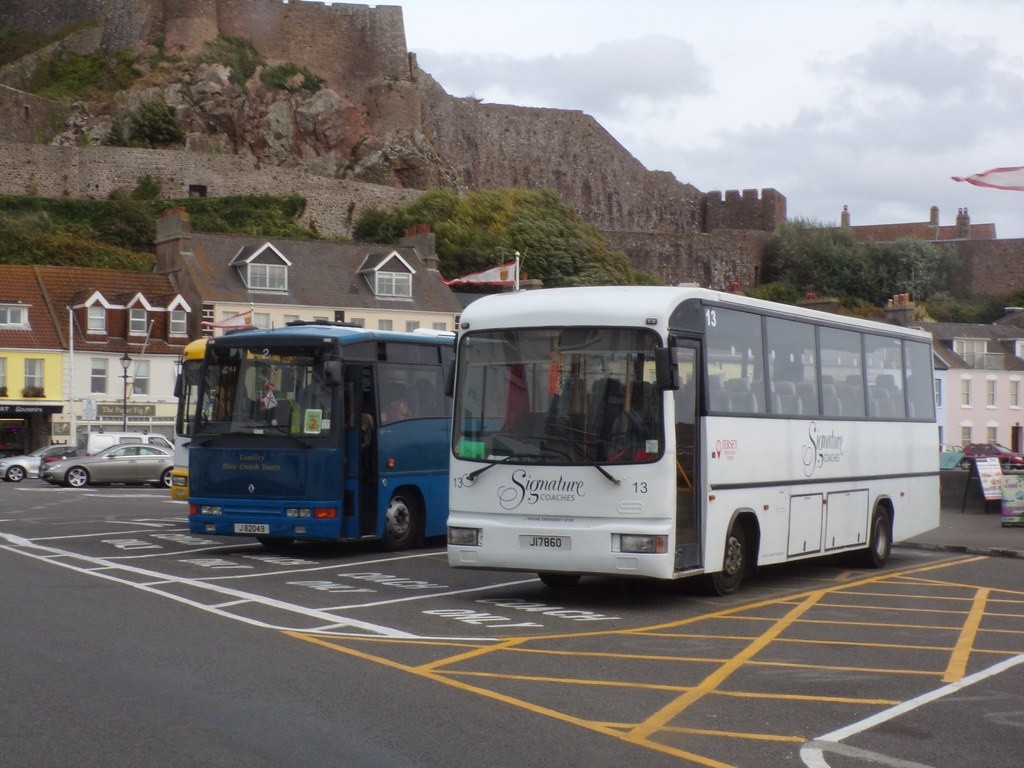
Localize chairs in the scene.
[587,374,915,446]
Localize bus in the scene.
[171,338,316,502]
[188,322,593,555]
[456,286,941,600]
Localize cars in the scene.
[0,443,77,482]
[40,444,174,489]
[40,446,87,463]
[960,443,1024,470]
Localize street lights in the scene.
[118,352,134,432]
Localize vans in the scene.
[86,432,174,456]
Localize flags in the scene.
[264,391,278,410]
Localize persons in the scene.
[238,384,255,409]
[389,396,410,420]
[348,401,374,447]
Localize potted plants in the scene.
[21,387,46,398]
[0,387,8,397]
